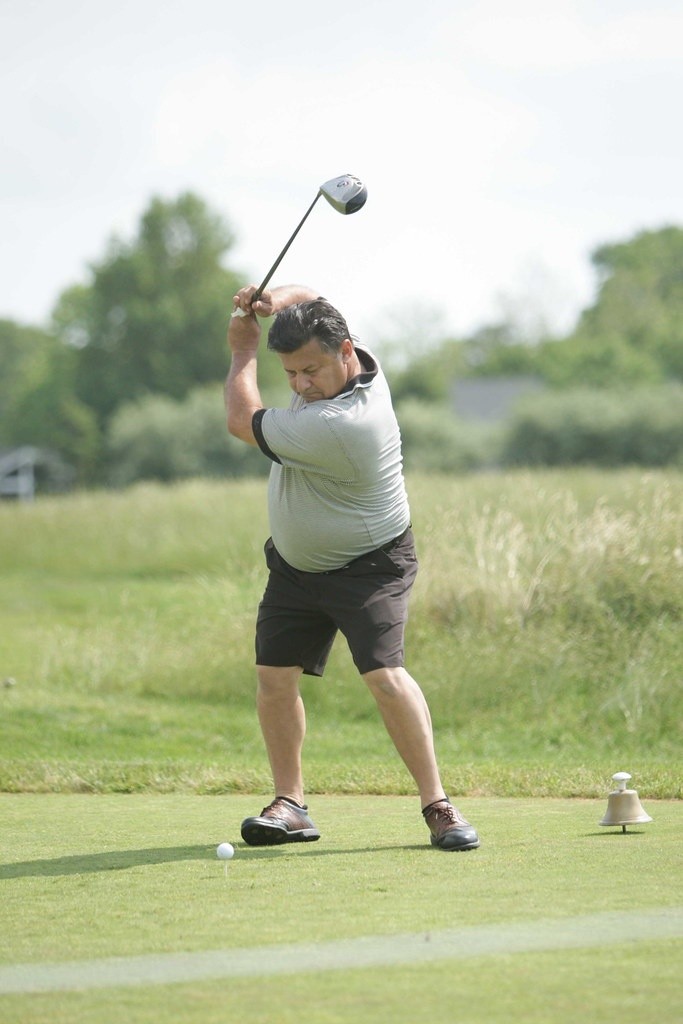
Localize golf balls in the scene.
[216,842,234,859]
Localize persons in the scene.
[222,283,480,852]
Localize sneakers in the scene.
[425,801,480,851]
[241,799,321,846]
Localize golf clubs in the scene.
[249,172,369,303]
